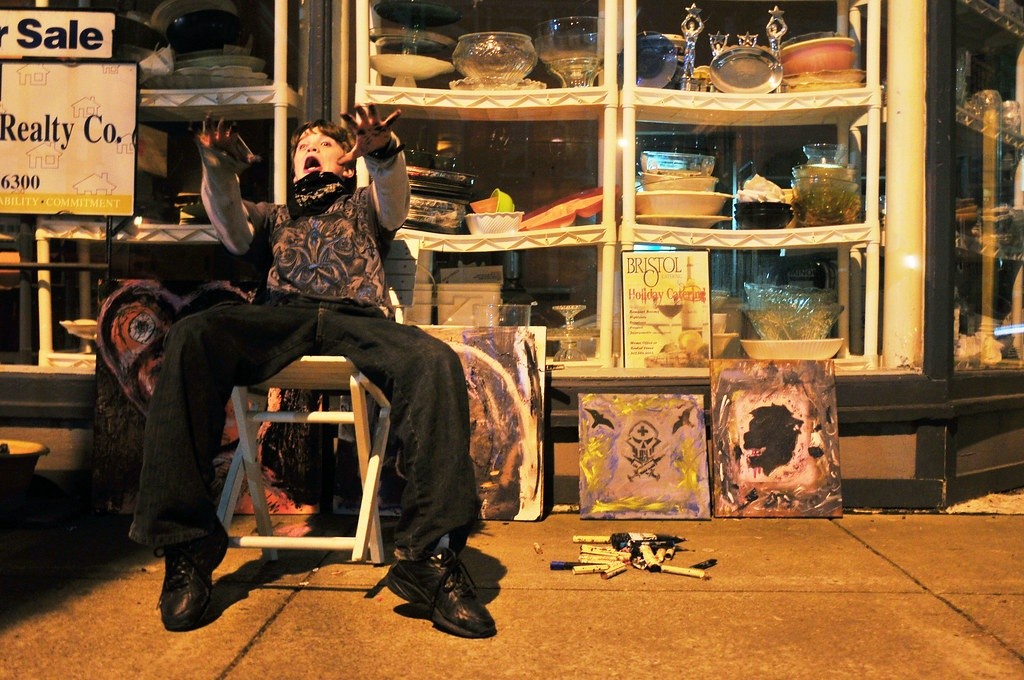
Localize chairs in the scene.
[209,282,399,567]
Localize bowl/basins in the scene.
[113,0,269,46]
[0,439,52,472]
[59,318,99,341]
[401,147,459,172]
[733,202,794,230]
[635,149,735,229]
[532,15,606,87]
[711,289,739,358]
[464,186,525,236]
[790,142,863,228]
[778,36,857,71]
[737,282,846,340]
[450,32,538,79]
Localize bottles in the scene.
[679,256,704,339]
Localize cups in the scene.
[473,304,531,326]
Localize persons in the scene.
[128,104,498,639]
[710,38,727,57]
[680,13,703,78]
[739,38,758,47]
[766,15,787,59]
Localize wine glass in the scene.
[655,302,684,351]
[551,305,587,362]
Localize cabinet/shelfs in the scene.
[0,0,1024,515]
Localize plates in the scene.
[448,76,547,91]
[619,30,678,88]
[709,46,783,94]
[782,69,867,89]
[169,48,273,87]
[547,357,604,370]
[739,338,846,359]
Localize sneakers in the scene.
[152,516,230,631]
[386,548,496,638]
